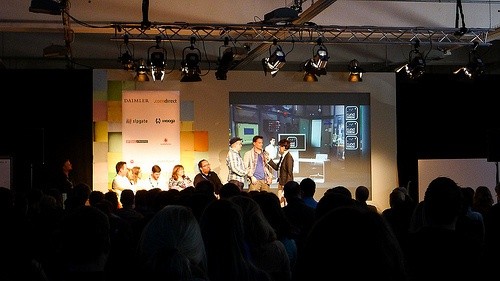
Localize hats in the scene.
[229,137,242,146]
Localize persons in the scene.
[143,165,169,191]
[243,135,273,192]
[169,165,195,192]
[0,163,500,281]
[225,137,251,193]
[56,157,75,194]
[194,159,224,199]
[263,139,294,206]
[265,137,278,160]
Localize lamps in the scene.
[452,55,488,79]
[395,40,426,79]
[262,39,286,79]
[348,58,364,82]
[215,36,235,81]
[302,37,330,82]
[179,36,203,83]
[118,34,167,82]
